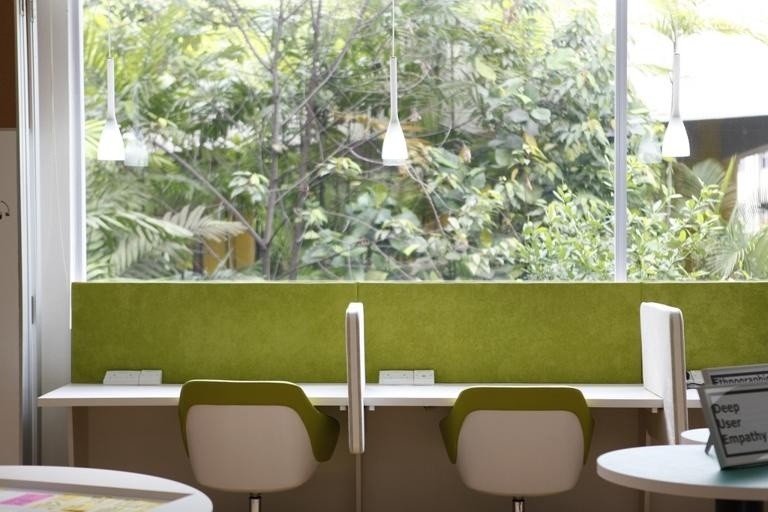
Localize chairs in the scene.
[178,379,341,512]
[439,387,595,511]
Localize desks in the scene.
[681,428,712,445]
[595,443,768,509]
[1,465,214,511]
[34,383,703,468]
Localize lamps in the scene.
[96,0,126,162]
[660,2,691,159]
[381,1,409,168]
